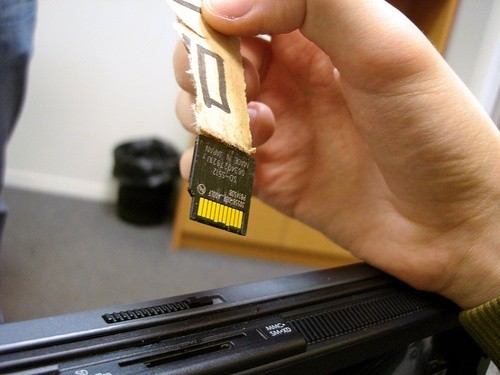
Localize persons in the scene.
[168,1,500,374]
